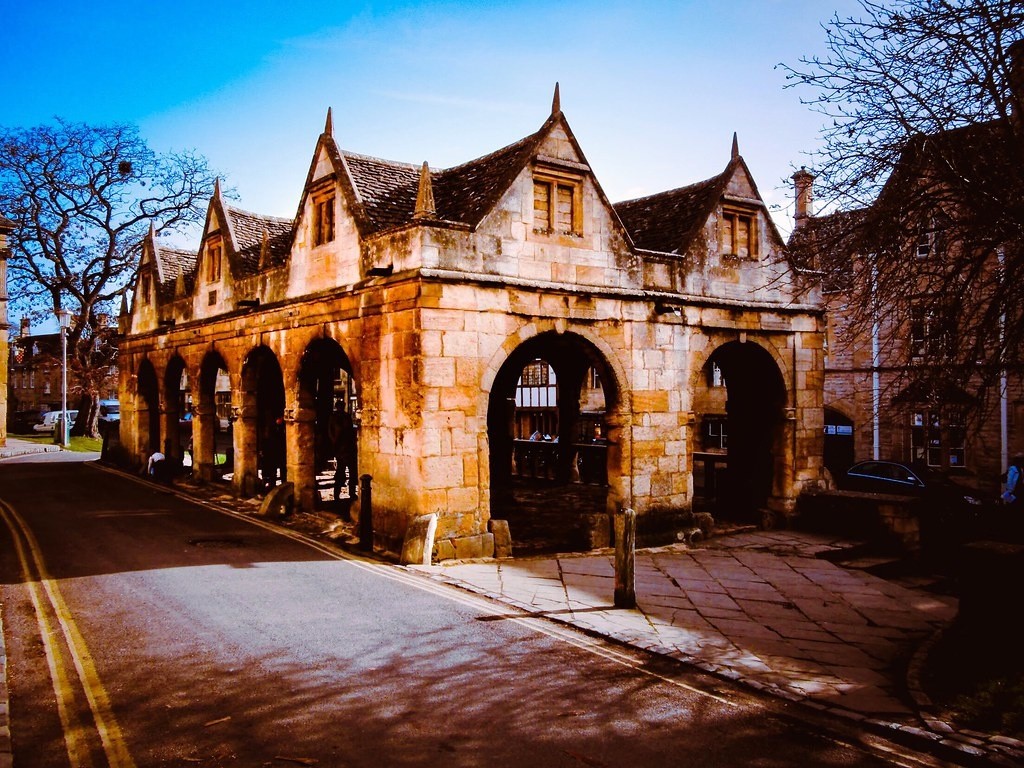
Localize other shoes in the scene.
[335,498,340,503]
[350,494,358,500]
[270,486,276,490]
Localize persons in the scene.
[1000,456,1024,503]
[147,448,167,482]
[256,390,357,504]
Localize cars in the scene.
[831,458,989,528]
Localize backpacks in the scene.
[1000,463,1022,483]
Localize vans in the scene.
[99,399,120,422]
[32,409,79,435]
